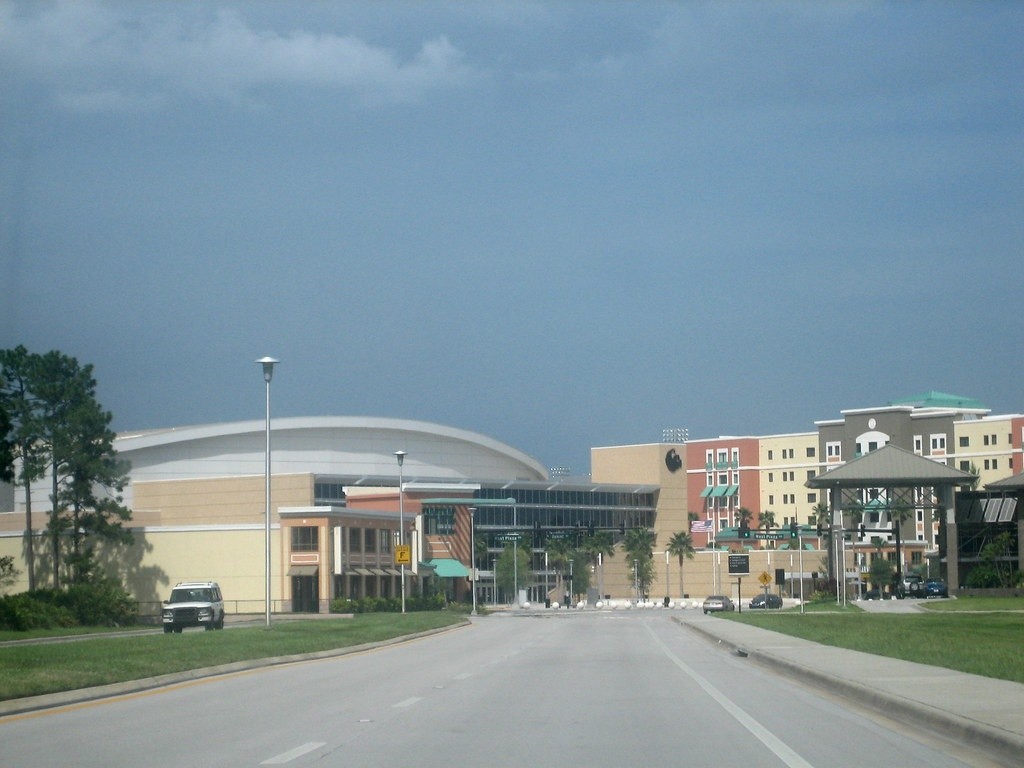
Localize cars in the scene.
[924,577,948,598]
[862,588,892,601]
[703,595,735,614]
[749,593,784,610]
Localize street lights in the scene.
[257,356,281,622]
[467,507,478,616]
[569,558,574,607]
[633,558,640,604]
[393,451,409,613]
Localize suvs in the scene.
[895,574,926,599]
[162,581,224,633]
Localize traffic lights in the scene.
[741,522,750,539]
[861,524,866,537]
[790,522,797,539]
